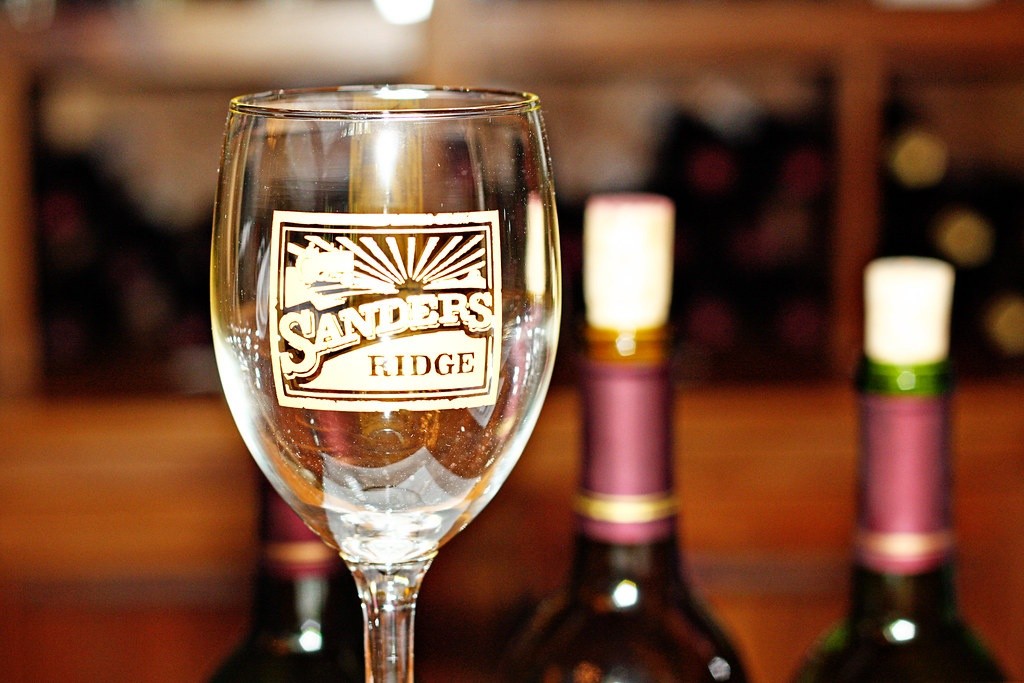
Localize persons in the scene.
[621,69,1024,382]
[19,66,272,384]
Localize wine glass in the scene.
[207,85,563,683]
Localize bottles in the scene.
[790,255,1010,683]
[481,192,753,683]
[211,174,364,683]
